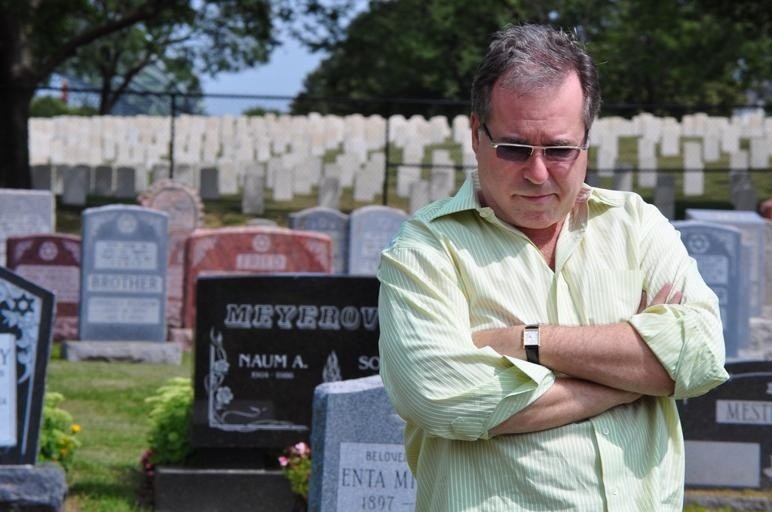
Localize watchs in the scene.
[523,323,540,364]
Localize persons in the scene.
[378,25,730,512]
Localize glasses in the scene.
[479,122,589,163]
